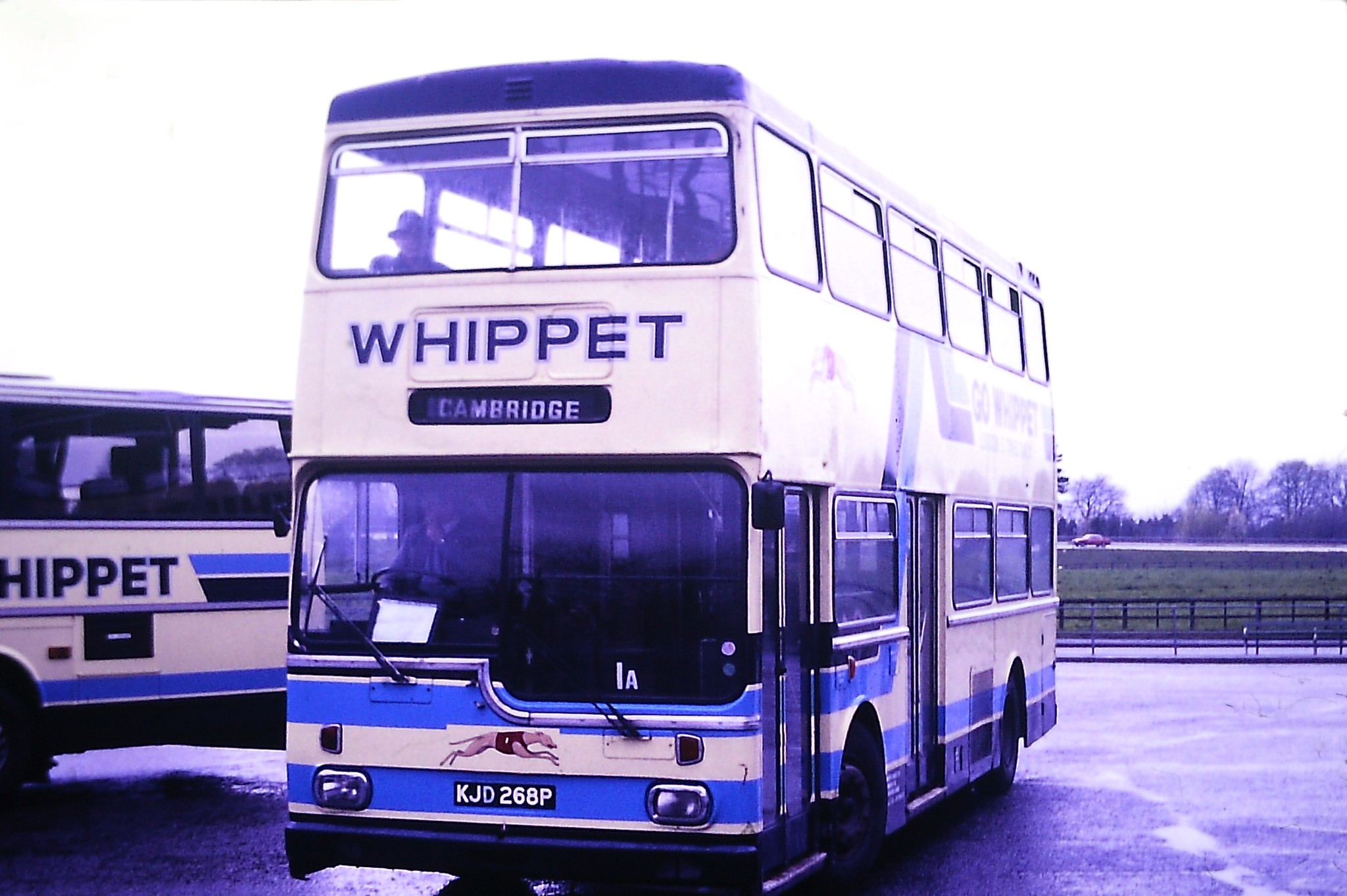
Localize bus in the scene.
[0,371,326,824]
[282,58,1062,896]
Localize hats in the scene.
[388,210,424,238]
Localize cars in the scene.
[1072,534,1111,547]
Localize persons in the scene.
[380,484,498,596]
[377,211,448,275]
